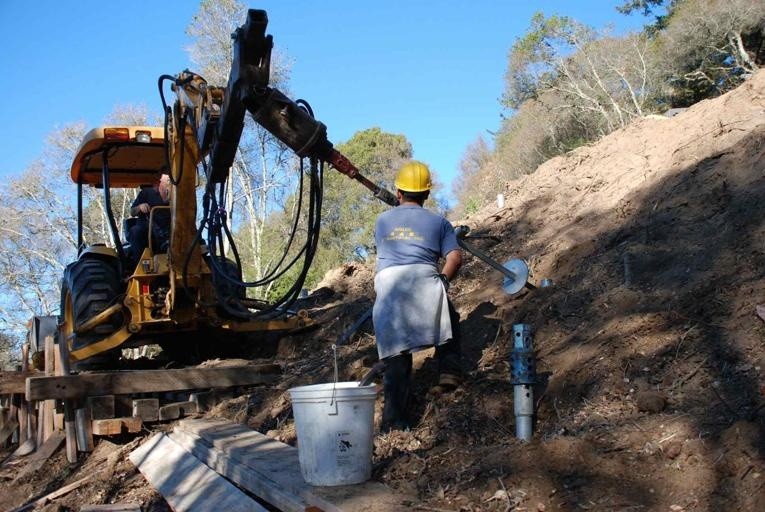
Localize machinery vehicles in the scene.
[53,8,549,374]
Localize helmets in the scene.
[395,160,432,193]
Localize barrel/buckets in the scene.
[287,343,378,485]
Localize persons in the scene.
[373,161,464,435]
[126,174,169,269]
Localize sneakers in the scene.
[438,374,460,388]
